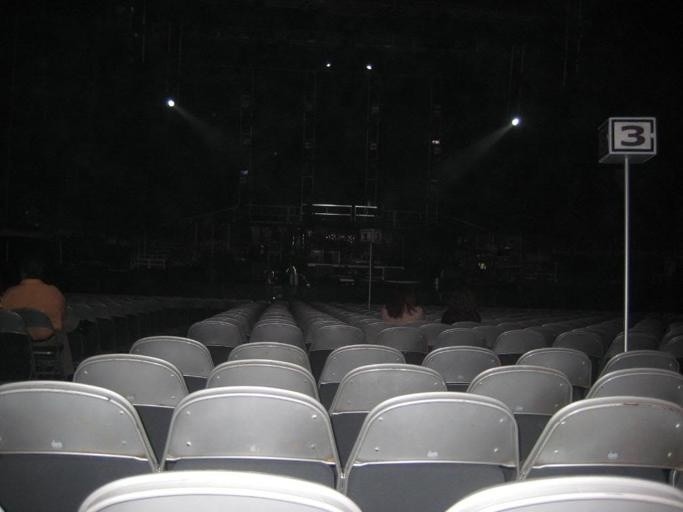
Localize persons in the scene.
[1,251,77,379]
[441,285,482,324]
[378,286,423,322]
